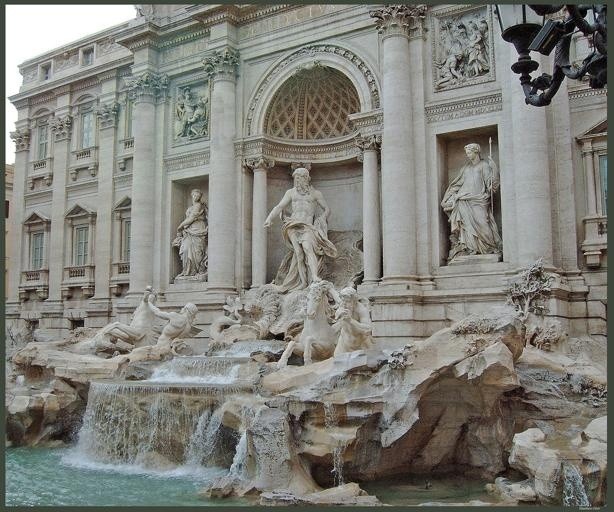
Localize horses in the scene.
[275,283,338,369]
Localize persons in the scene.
[111,295,203,367]
[436,13,488,93]
[171,188,208,279]
[262,167,337,292]
[441,142,503,261]
[333,289,374,356]
[174,87,208,141]
